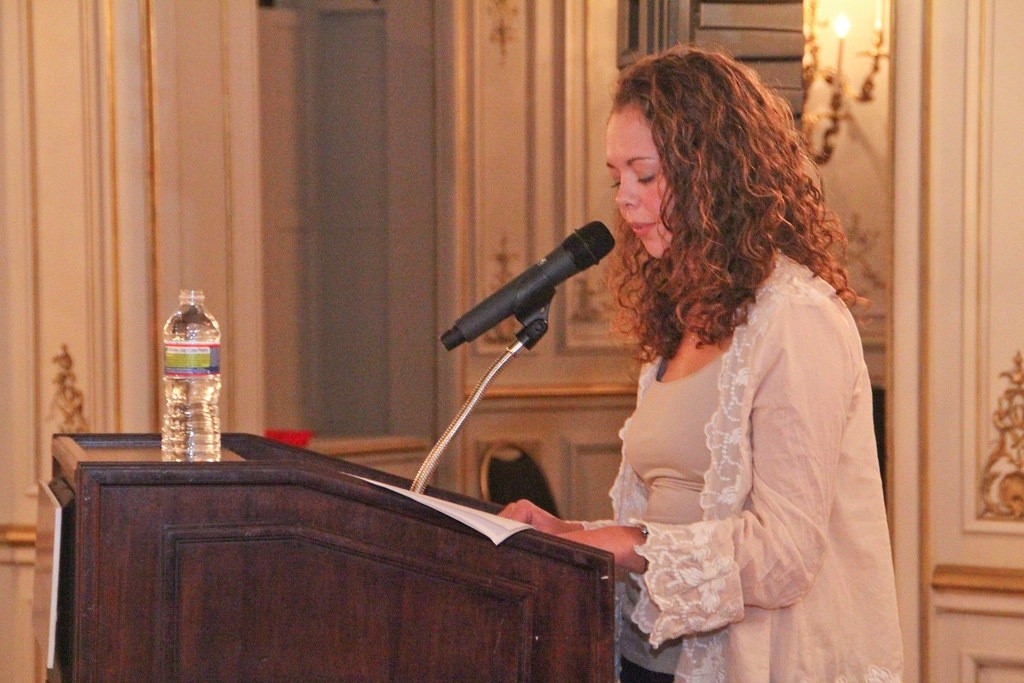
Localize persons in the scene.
[498,44,903,683]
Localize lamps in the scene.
[799,6,890,166]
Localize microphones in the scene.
[438,221,615,352]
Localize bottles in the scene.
[161,290,221,463]
